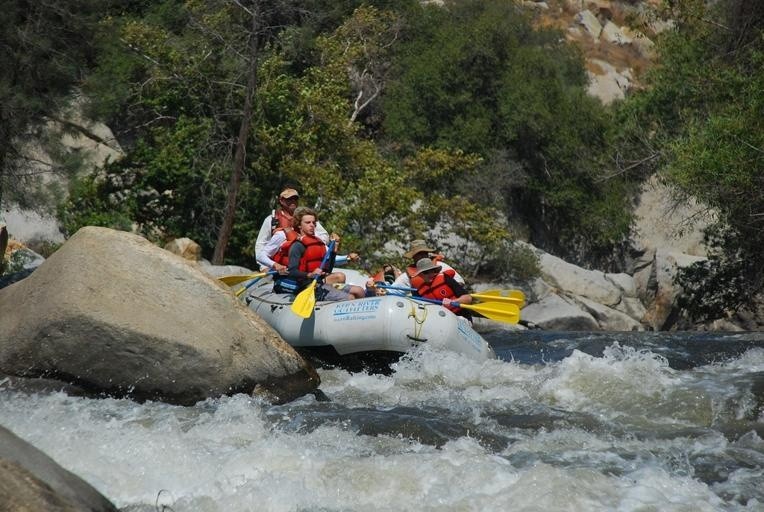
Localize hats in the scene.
[411,258,442,278]
[279,189,300,200]
[402,240,436,259]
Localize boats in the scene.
[235,273,498,382]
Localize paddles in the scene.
[372,284,525,310]
[217,257,351,287]
[385,292,520,324]
[292,240,336,319]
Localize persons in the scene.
[252,185,339,273]
[407,257,473,320]
[375,239,465,296]
[255,206,358,294]
[287,205,365,302]
